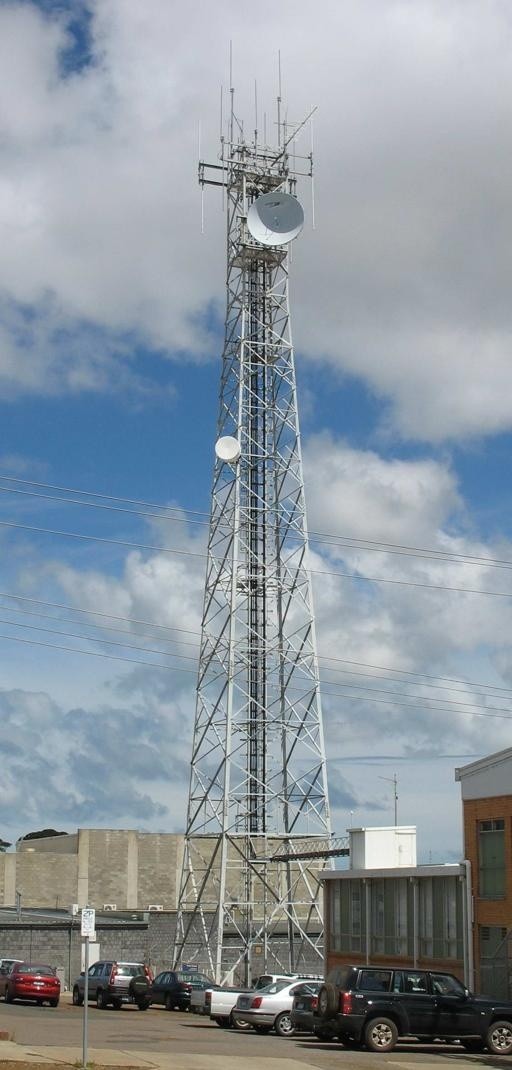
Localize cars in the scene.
[233,979,325,1036]
[152,971,221,1011]
[290,993,340,1039]
[0,959,60,1006]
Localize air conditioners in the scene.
[102,904,116,911]
[148,904,164,911]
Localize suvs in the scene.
[73,959,154,1011]
[312,964,512,1056]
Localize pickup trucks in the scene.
[188,974,294,1028]
